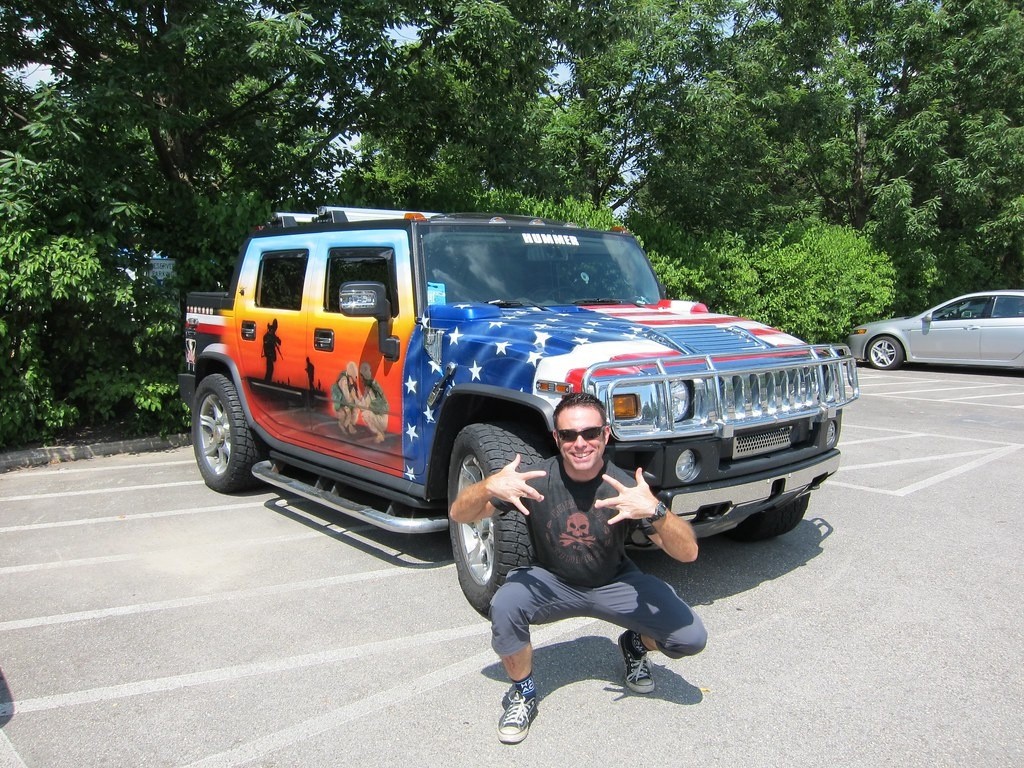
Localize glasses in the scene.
[558,426,604,441]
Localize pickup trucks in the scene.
[181,206,861,617]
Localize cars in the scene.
[848,291,1024,371]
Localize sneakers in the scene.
[497,690,537,743]
[618,630,654,693]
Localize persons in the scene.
[449,390,709,745]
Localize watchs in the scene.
[646,501,667,523]
